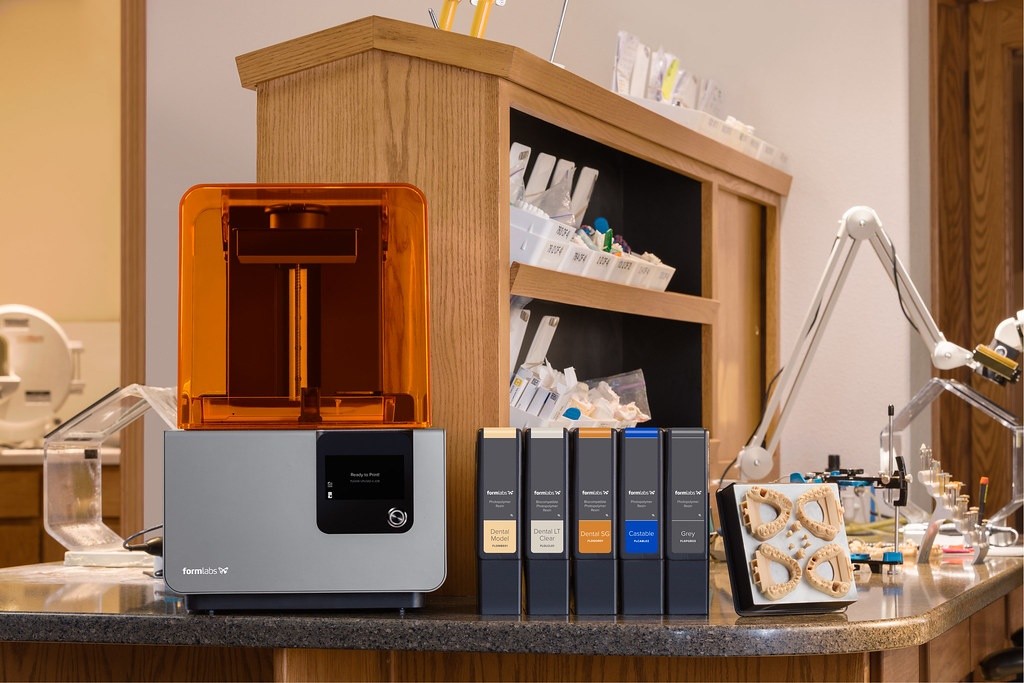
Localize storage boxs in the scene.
[616,92,789,171]
[511,202,676,293]
[509,404,637,431]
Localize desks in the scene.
[2,552,1024,683]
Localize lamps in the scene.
[711,206,1024,530]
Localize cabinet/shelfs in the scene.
[0,449,121,570]
[235,16,793,603]
